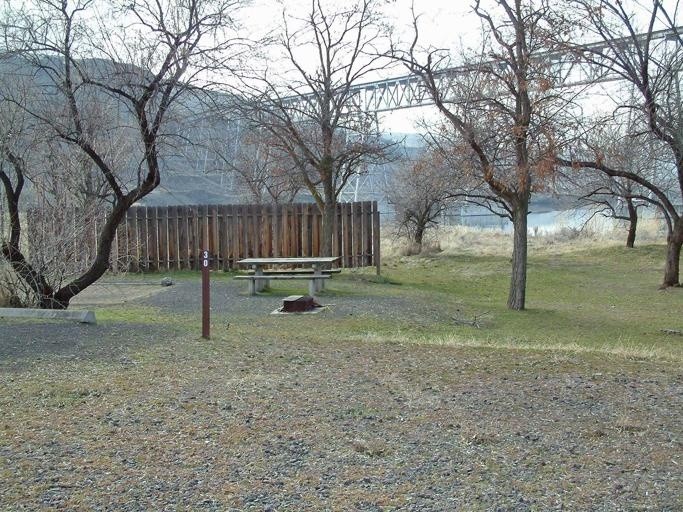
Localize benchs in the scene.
[248,269,341,289]
[234,274,332,295]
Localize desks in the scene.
[237,258,342,291]
